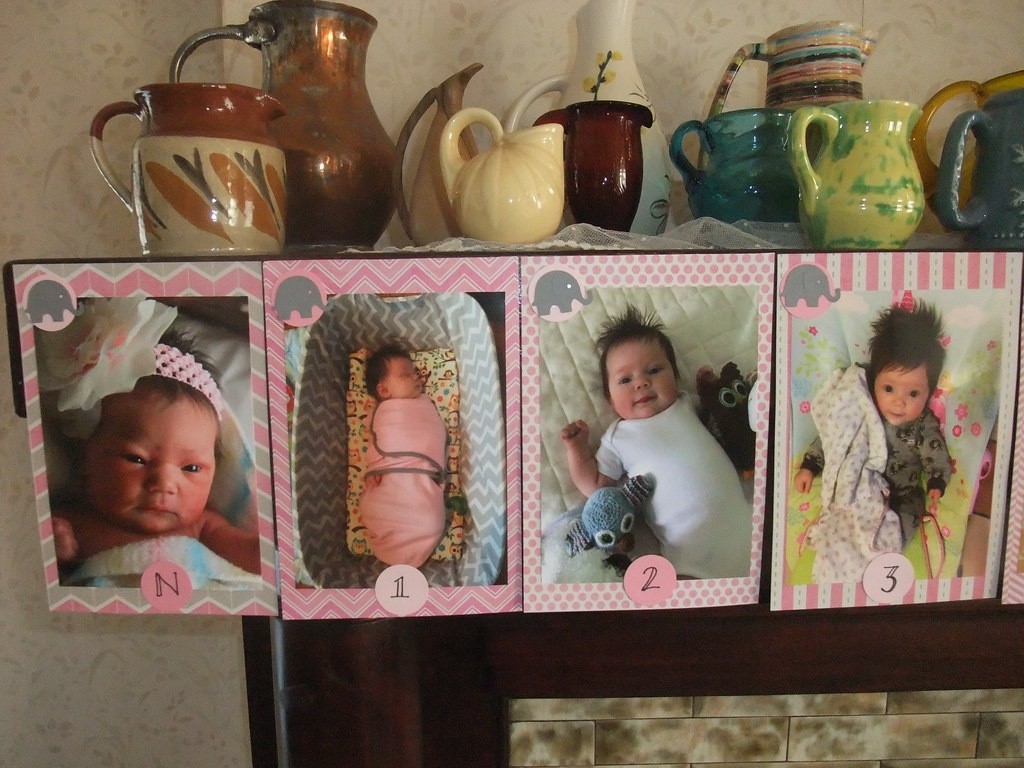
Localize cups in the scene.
[669,107,801,223]
[908,70,1024,250]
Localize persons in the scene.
[52,328,263,587]
[794,297,953,551]
[560,306,753,579]
[357,342,449,567]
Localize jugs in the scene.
[395,61,485,246]
[439,108,565,243]
[530,99,653,232]
[91,82,288,254]
[786,99,924,251]
[696,19,882,172]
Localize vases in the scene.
[89,0,1024,251]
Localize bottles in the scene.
[500,0,667,237]
[170,1,396,249]
